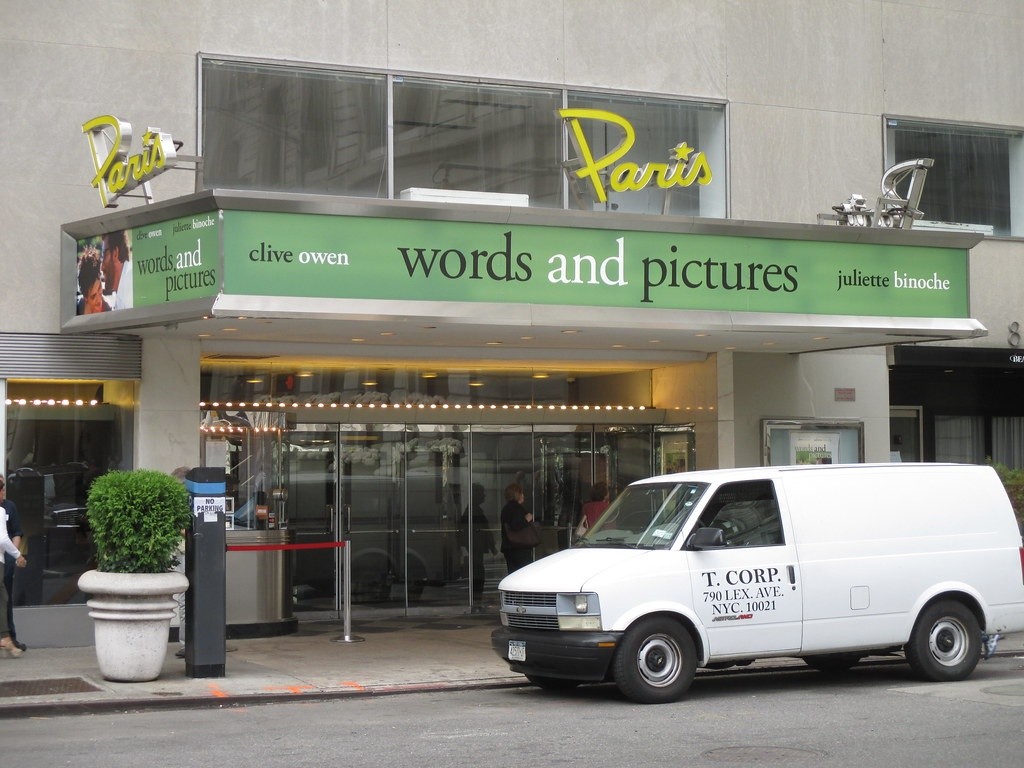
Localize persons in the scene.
[0,474,27,659]
[582,482,617,531]
[459,484,497,615]
[77,229,133,314]
[501,483,534,574]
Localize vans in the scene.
[232,474,458,601]
[491,461,1024,704]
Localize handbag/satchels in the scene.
[505,523,541,548]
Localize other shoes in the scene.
[13,641,26,651]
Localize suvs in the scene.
[5,461,106,578]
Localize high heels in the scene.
[0,639,24,658]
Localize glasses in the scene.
[102,247,111,261]
